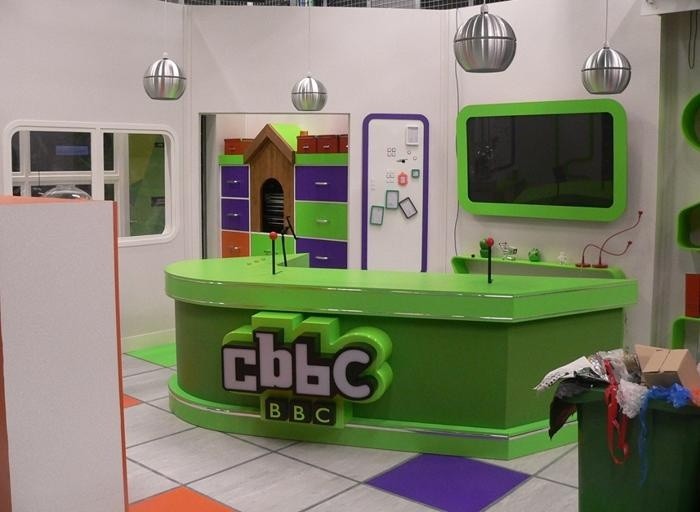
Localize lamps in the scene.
[453,0,631,94]
[142,0,188,99]
[290,0,328,112]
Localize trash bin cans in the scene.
[562,386,699,511]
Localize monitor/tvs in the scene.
[455,97,628,223]
